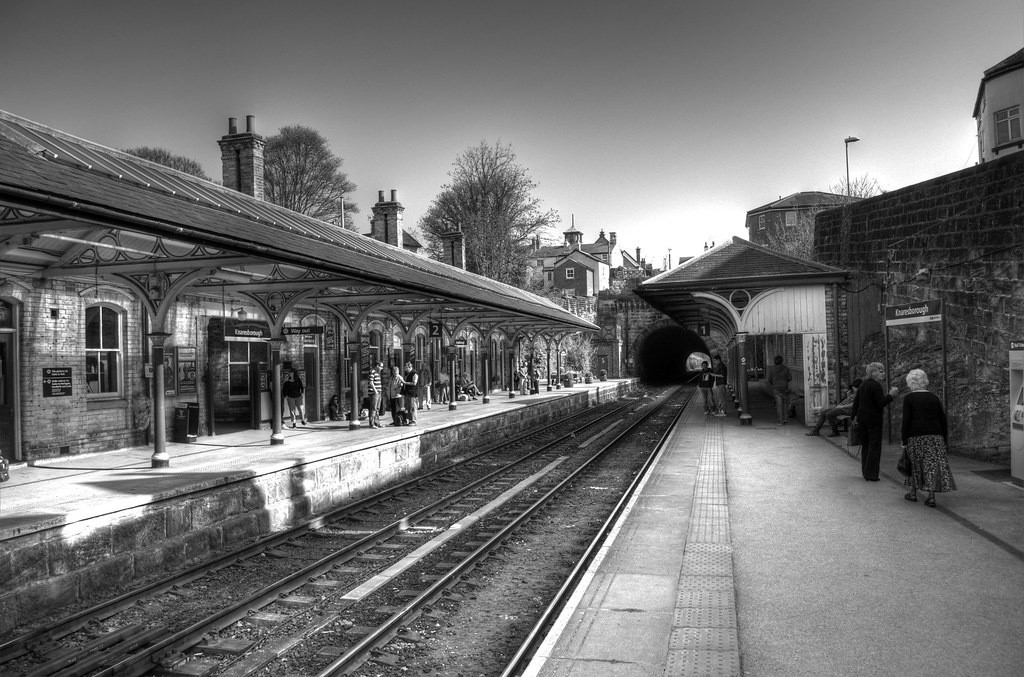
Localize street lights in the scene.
[845,136,860,202]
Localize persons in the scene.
[896,369,958,507]
[519,362,541,395]
[767,356,792,426]
[804,379,863,436]
[697,355,727,417]
[850,362,899,482]
[368,360,432,428]
[268,362,307,429]
[328,394,347,421]
[439,367,483,405]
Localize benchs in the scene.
[837,415,851,432]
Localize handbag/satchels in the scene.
[393,411,411,426]
[847,423,863,447]
[897,447,912,477]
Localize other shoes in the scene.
[828,432,840,437]
[776,422,783,425]
[372,425,378,429]
[714,413,727,416]
[418,408,423,410]
[473,398,478,400]
[705,411,715,415]
[440,402,448,405]
[477,393,483,396]
[784,418,788,422]
[282,424,289,429]
[410,421,416,426]
[293,423,296,428]
[805,431,819,436]
[429,408,432,410]
[390,422,394,425]
[924,497,936,508]
[905,493,917,502]
[865,478,880,481]
[301,420,307,425]
[379,424,385,427]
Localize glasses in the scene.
[381,366,384,367]
